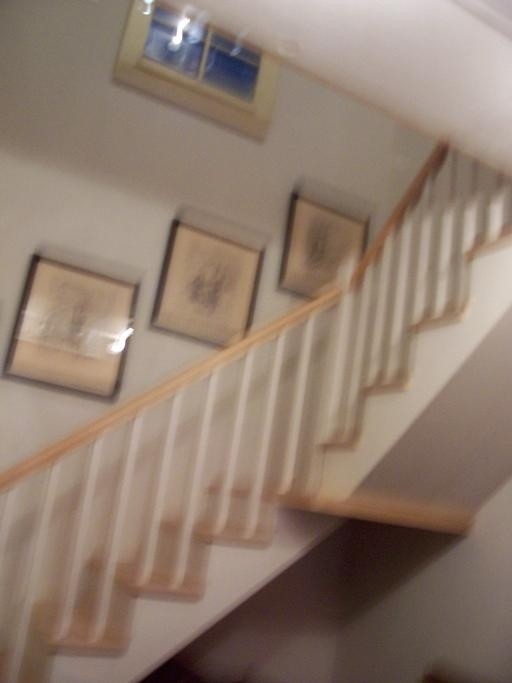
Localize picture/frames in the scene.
[3,254,140,400]
[279,192,371,299]
[151,219,265,350]
